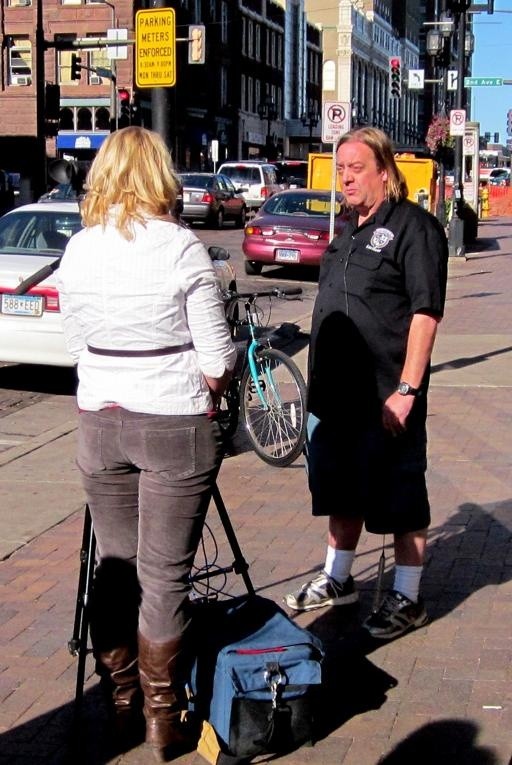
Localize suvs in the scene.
[214,159,286,213]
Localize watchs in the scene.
[398,382,417,396]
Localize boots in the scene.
[138,627,190,761]
[86,610,143,736]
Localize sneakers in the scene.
[361,591,429,639]
[283,572,358,610]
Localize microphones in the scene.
[347,239,357,255]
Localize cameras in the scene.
[169,184,184,218]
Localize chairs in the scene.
[36,230,66,251]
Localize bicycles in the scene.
[210,288,312,467]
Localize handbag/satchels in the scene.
[182,595,325,755]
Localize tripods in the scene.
[68,479,256,714]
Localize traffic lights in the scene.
[387,55,403,98]
[115,85,133,136]
[69,52,82,81]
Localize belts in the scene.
[87,343,194,358]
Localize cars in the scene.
[242,190,354,276]
[0,202,91,369]
[170,172,247,229]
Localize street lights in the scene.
[425,7,475,231]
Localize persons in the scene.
[56,125,240,763]
[282,126,449,639]
